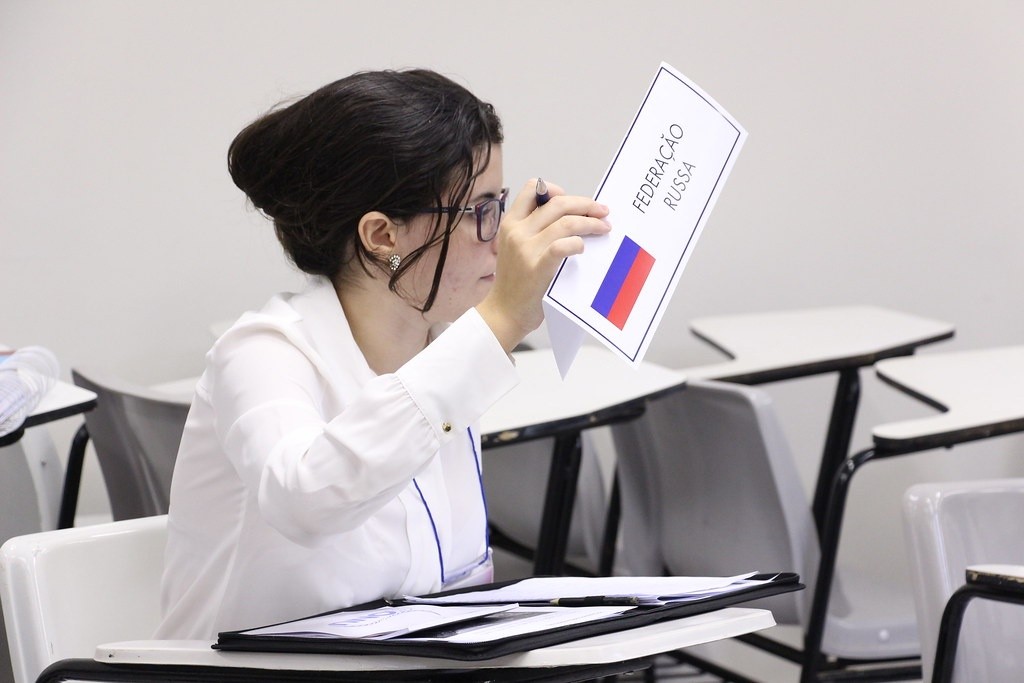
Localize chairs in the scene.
[1,302,1024,683]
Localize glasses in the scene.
[382,188,508,242]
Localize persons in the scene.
[161,66,612,642]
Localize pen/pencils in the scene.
[549,597,637,606]
[535,177,549,207]
